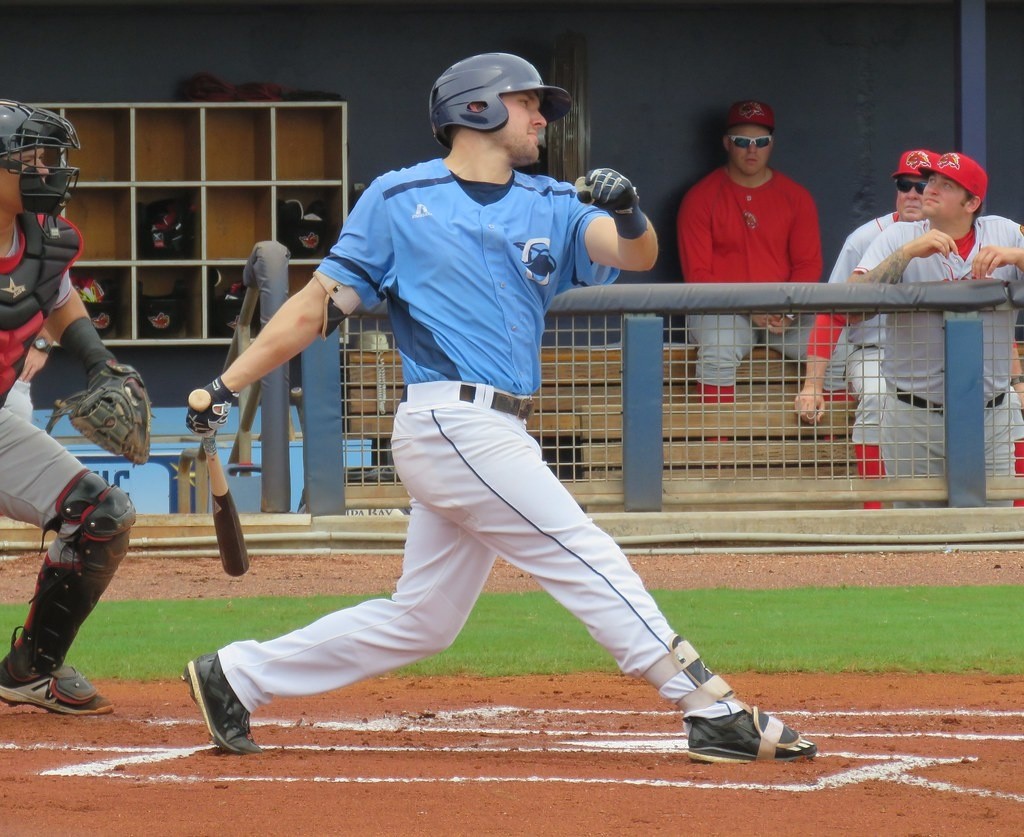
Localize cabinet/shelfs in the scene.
[21,99,349,346]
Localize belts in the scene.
[896,388,1004,415]
[401,385,533,419]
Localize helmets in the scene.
[428,52,572,150]
[0,98,36,156]
[140,199,193,256]
[280,199,329,257]
[211,273,244,336]
[78,281,120,336]
[138,277,186,340]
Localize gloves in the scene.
[185,376,238,434]
[579,168,640,216]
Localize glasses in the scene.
[727,135,772,148]
[896,181,927,195]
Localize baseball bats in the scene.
[188,388,252,576]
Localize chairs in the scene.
[208,462,263,515]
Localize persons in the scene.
[0,102,151,716]
[793,147,1024,507]
[186,52,818,767]
[681,101,855,466]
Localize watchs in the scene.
[31,338,52,355]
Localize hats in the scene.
[726,101,775,132]
[891,150,934,176]
[919,152,987,200]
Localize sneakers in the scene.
[0,650,114,714]
[688,706,817,764]
[182,653,262,754]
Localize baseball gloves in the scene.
[67,359,150,466]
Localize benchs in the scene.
[345,340,1023,509]
